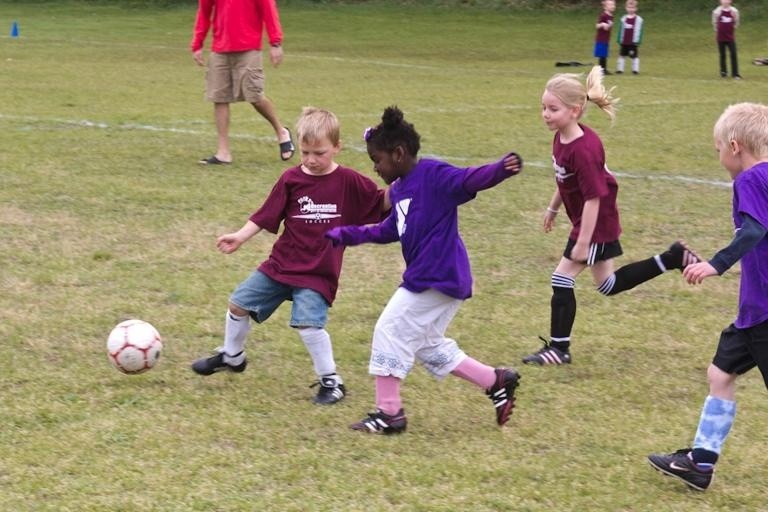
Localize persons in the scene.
[189,104,393,406]
[710,0,744,81]
[648,100,768,493]
[324,107,523,437]
[613,0,646,74]
[190,0,296,165]
[522,70,705,368]
[592,0,616,75]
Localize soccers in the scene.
[106,319,162,376]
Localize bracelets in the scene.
[546,206,559,213]
[271,43,282,48]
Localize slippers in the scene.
[279,127,294,160]
[203,155,232,166]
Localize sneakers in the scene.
[191,353,246,375]
[351,409,406,434]
[670,240,702,271]
[486,368,520,426]
[523,336,570,365]
[309,373,346,404]
[647,448,713,491]
